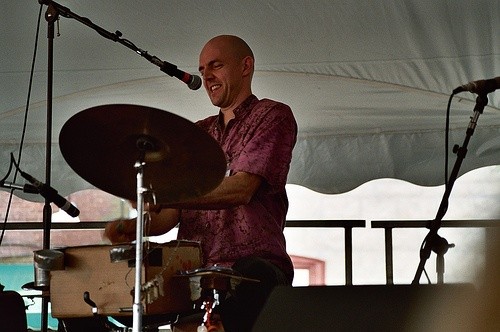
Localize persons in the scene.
[104,34,297,331]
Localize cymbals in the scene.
[174,258,266,284]
[59,103,229,201]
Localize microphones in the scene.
[453,77,500,94]
[21,171,80,217]
[150,56,202,90]
